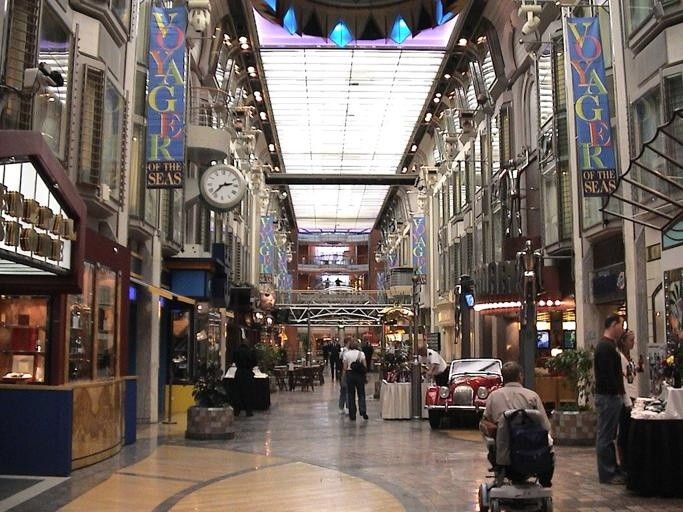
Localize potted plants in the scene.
[184,369,236,439]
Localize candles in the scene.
[549,345,597,444]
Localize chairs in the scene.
[271,357,328,392]
[381,380,435,421]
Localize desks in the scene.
[221,373,270,412]
[620,398,683,501]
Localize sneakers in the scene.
[339,408,368,421]
[599,465,629,485]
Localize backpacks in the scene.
[503,406,556,490]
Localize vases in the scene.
[386,368,409,383]
[665,385,683,418]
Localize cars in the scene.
[424,358,503,429]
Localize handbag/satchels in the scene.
[350,350,367,373]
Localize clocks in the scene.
[198,163,247,210]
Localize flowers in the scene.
[382,348,412,370]
[661,345,683,387]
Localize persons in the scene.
[334,278,342,294]
[336,337,353,415]
[329,336,341,380]
[592,314,629,485]
[340,341,368,420]
[481,361,550,478]
[231,339,257,416]
[417,344,448,387]
[322,278,330,294]
[615,329,643,474]
[362,341,373,374]
